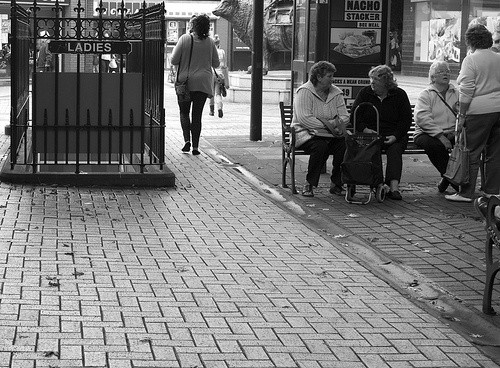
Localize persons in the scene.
[37,39,56,72]
[93,53,120,73]
[445,25,500,201]
[209,35,228,119]
[413,60,459,202]
[351,65,412,203]
[171,15,219,156]
[289,61,350,198]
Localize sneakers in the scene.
[182,142,191,152]
[388,190,402,200]
[438,178,450,192]
[445,194,472,201]
[475,196,500,247]
[192,150,201,155]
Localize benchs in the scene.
[280,102,454,194]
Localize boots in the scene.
[209,105,214,115]
[218,109,223,117]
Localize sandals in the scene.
[329,184,346,195]
[302,184,313,196]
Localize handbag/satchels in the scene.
[443,126,470,184]
[176,82,190,102]
